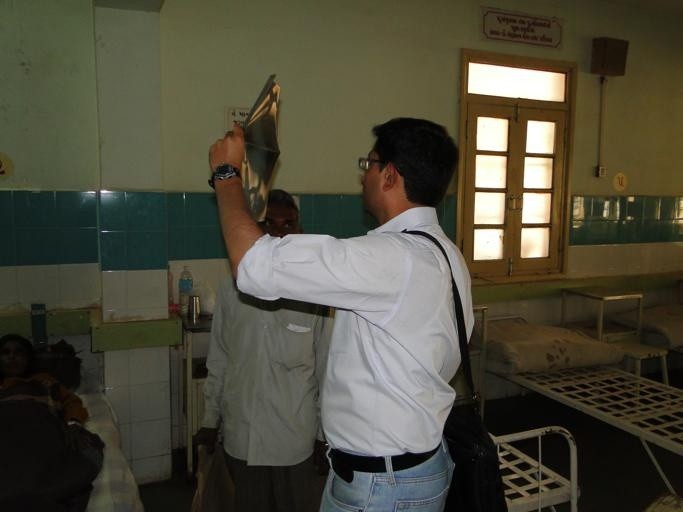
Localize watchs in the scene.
[208,164,240,190]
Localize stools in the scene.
[560,285,644,350]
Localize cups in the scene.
[188,295,202,320]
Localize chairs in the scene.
[444,405,580,512]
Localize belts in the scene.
[327,444,441,486]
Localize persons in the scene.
[191,188,335,512]
[0,333,84,512]
[208,116,475,512]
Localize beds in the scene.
[470,312,683,461]
[0,311,144,511]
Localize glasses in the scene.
[357,155,405,176]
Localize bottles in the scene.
[178,264,194,316]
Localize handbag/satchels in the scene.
[402,223,510,512]
